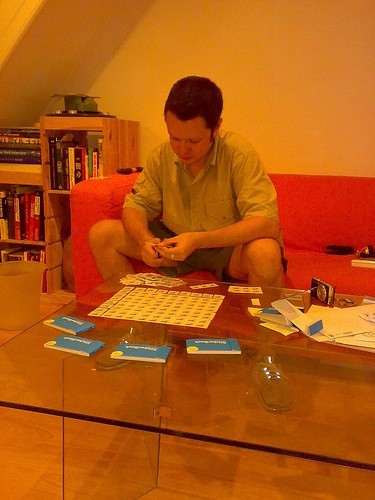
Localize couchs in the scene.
[70,173,375,384]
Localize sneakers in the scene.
[93,326,154,371]
[251,356,295,411]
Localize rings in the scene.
[171,254,175,260]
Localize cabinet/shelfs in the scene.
[40,116,140,295]
[0,163,44,266]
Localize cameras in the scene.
[310,276,335,306]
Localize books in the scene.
[0,127,41,165]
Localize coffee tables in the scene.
[0,272,375,500]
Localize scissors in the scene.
[152,245,163,258]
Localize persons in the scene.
[89,76,294,412]
[0,188,45,242]
[0,245,47,293]
[48,136,104,191]
